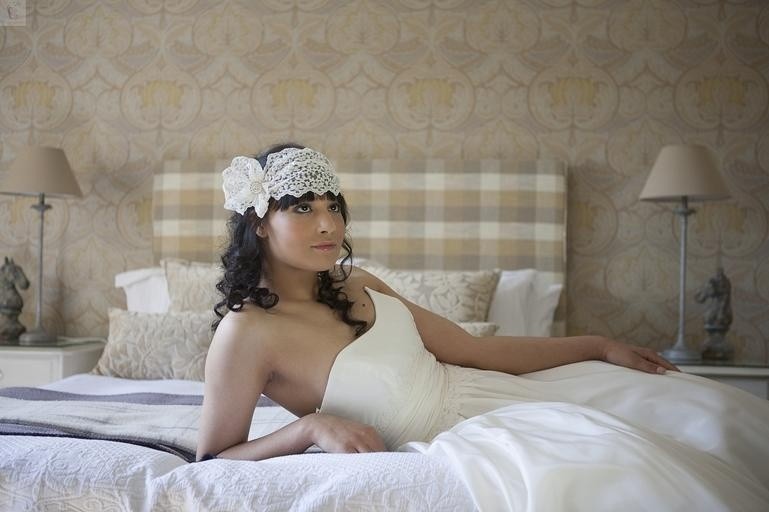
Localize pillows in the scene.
[161,254,503,322]
[446,320,499,343]
[88,307,223,381]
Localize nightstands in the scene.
[1,339,104,385]
[675,366,764,395]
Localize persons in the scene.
[191,144,769,512]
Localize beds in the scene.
[1,152,571,512]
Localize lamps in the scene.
[635,141,734,371]
[1,144,87,349]
